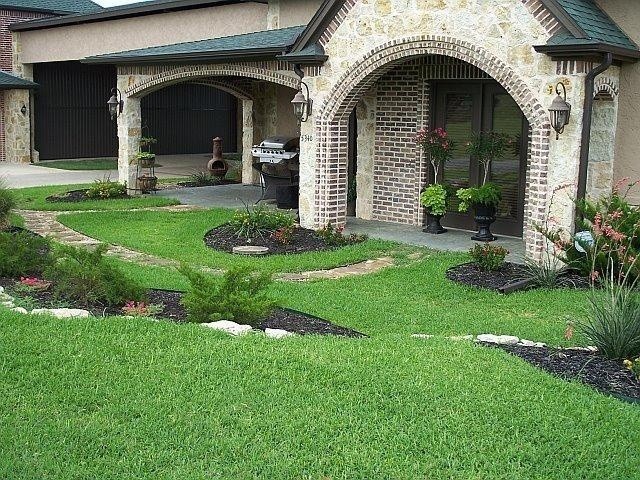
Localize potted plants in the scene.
[457,182,507,243]
[133,137,161,192]
[419,182,449,235]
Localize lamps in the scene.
[105,87,126,124]
[290,80,314,124]
[20,103,26,117]
[546,82,573,144]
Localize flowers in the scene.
[411,127,457,183]
